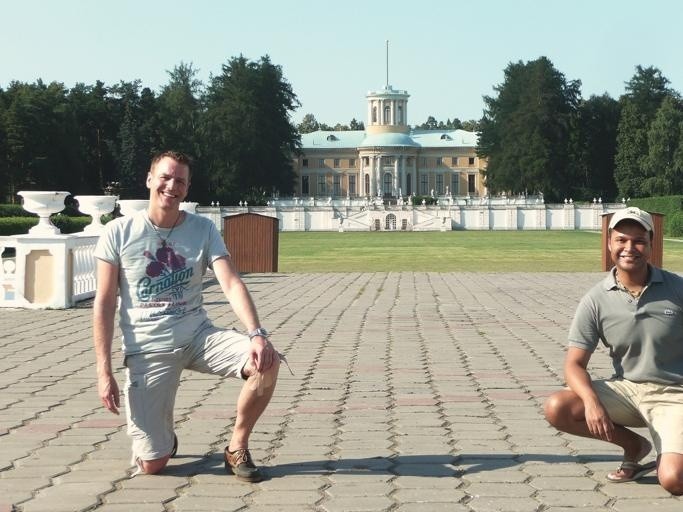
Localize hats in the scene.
[608,207,654,233]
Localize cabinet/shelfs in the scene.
[221,211,279,273]
[599,210,666,272]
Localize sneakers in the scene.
[169,435,177,456]
[224,446,262,482]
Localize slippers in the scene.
[605,460,657,483]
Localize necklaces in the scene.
[145,211,181,252]
[612,270,650,300]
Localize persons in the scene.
[90,150,280,482]
[541,206,683,496]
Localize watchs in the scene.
[248,327,268,341]
